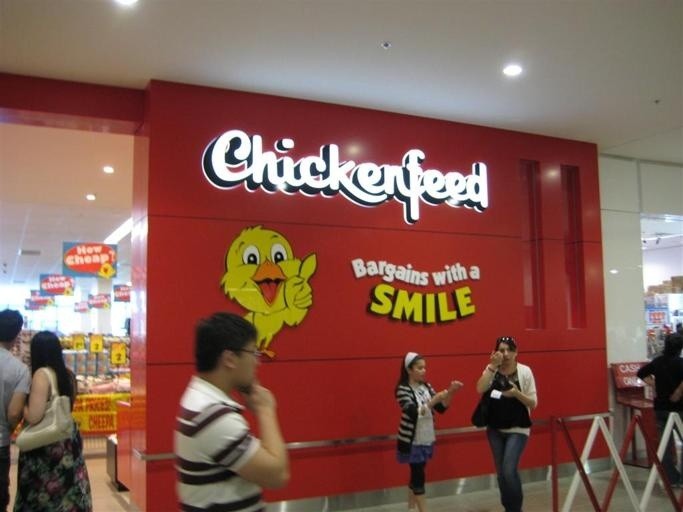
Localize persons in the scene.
[676,323,683,333]
[636,333,683,487]
[476,335,538,511]
[125,319,130,335]
[0,310,32,511]
[85,333,92,351]
[395,353,464,512]
[13,331,93,511]
[173,311,290,512]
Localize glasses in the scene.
[224,348,262,357]
[498,337,511,342]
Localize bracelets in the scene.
[482,362,499,379]
[429,399,434,406]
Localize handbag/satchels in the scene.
[471,392,489,426]
[16,396,74,452]
